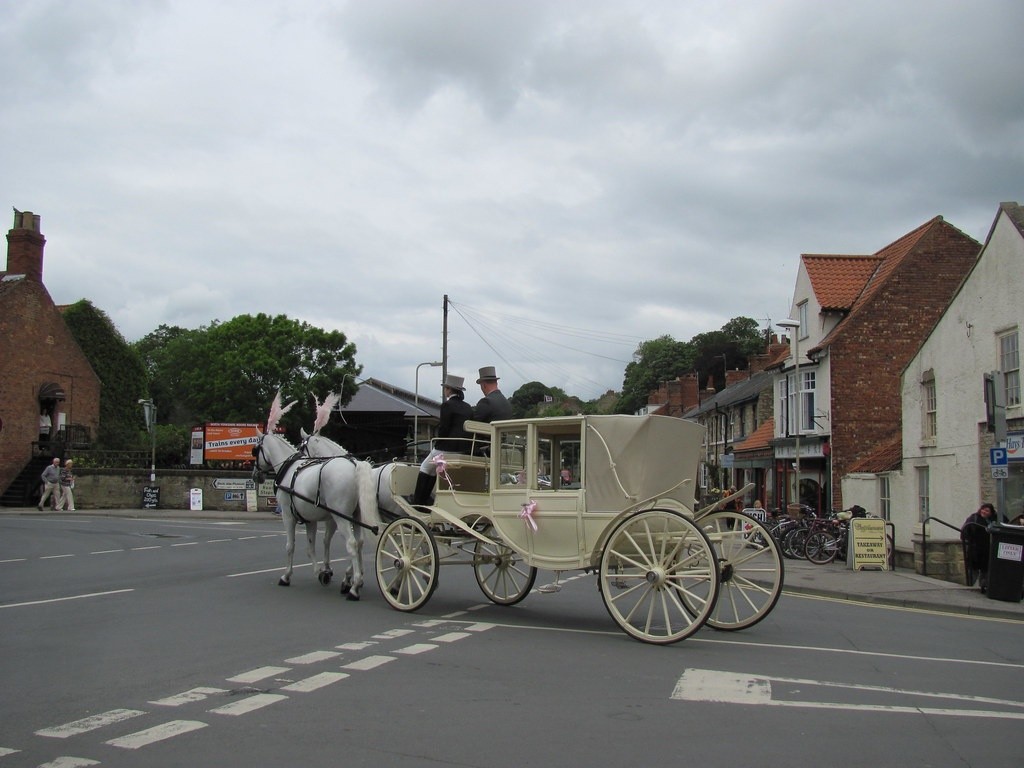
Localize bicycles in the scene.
[749,505,894,564]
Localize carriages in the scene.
[252,390,786,646]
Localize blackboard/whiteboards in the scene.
[142,486,161,509]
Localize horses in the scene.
[252,425,439,601]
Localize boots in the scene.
[414,471,436,506]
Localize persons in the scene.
[40,407,52,450]
[60,460,76,511]
[960,503,1009,593]
[37,458,60,512]
[474,366,512,456]
[407,375,474,509]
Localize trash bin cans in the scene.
[985,519,1024,603]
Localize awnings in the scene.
[40,381,65,401]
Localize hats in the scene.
[476,366,500,383]
[441,375,467,391]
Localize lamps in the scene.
[776,318,800,327]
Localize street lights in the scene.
[776,320,800,504]
[414,362,444,463]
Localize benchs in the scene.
[435,421,517,465]
